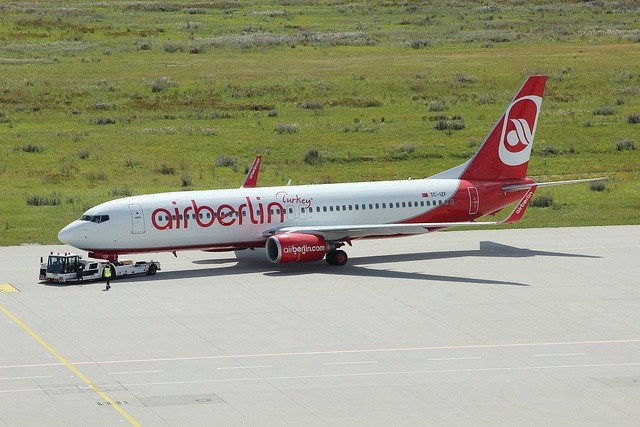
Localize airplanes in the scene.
[58,75,606,266]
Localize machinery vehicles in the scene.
[39,251,161,283]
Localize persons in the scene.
[103,263,111,290]
[77,264,83,281]
